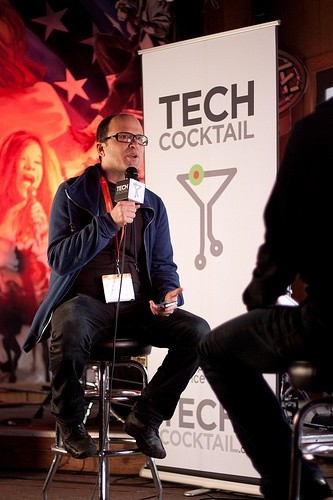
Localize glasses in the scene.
[101,132,148,146]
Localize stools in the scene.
[39,330,162,500]
[268,356,333,500]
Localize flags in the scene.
[4,1,172,123]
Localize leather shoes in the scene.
[123,414,166,459]
[58,422,96,460]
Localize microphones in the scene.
[27,186,43,247]
[114,167,146,224]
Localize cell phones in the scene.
[157,300,179,309]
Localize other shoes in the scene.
[264,478,333,500]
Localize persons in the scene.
[72,0,171,151]
[198,96,333,499]
[24,113,210,459]
[0,130,52,305]
